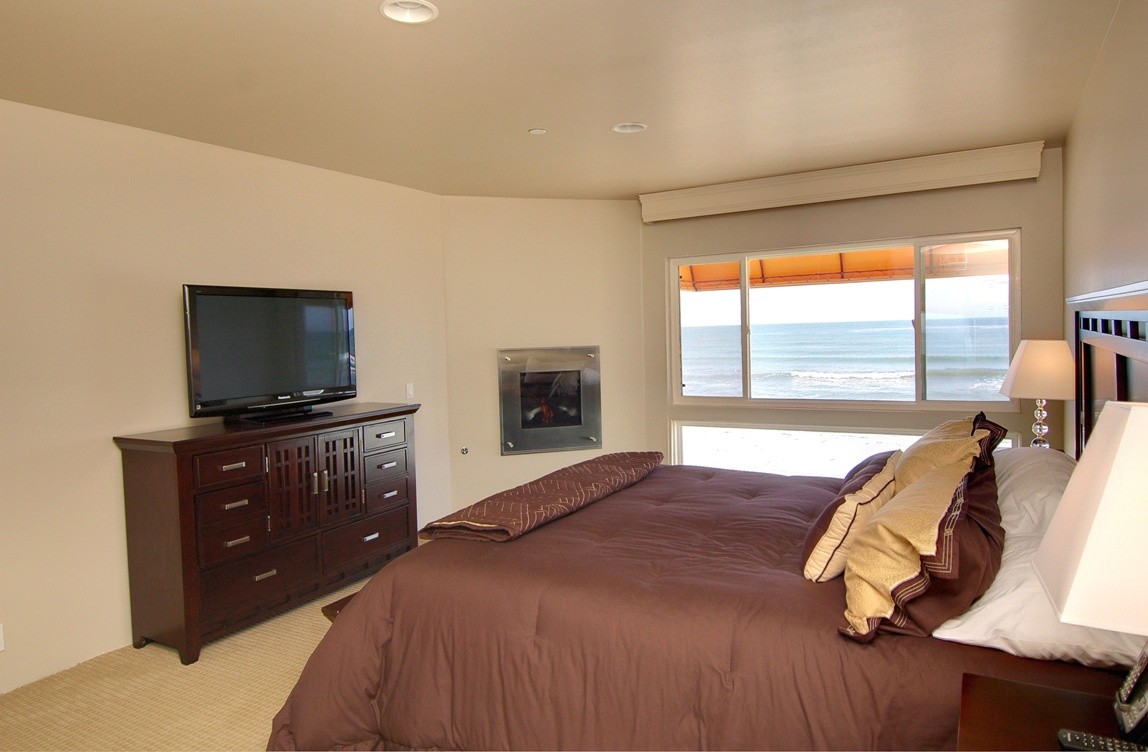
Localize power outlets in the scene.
[406,382,414,400]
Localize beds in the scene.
[268,278,1148,752]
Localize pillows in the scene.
[805,412,1147,669]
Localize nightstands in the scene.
[956,670,1148,752]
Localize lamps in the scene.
[1000,338,1077,448]
[1034,399,1148,671]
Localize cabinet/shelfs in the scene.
[113,401,429,660]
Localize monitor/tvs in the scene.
[182,284,357,428]
